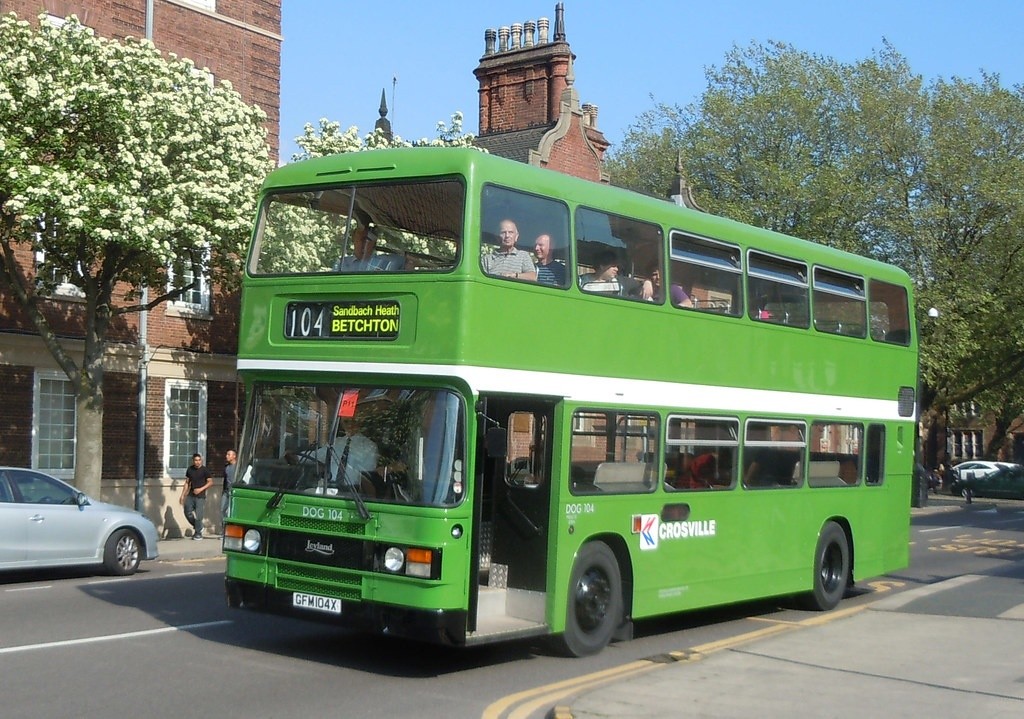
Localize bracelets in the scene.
[516,272,518,278]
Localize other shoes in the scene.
[192,533,203,540]
[218,537,223,540]
[199,528,205,533]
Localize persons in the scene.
[578,251,653,300]
[647,262,693,308]
[220,451,237,538]
[332,228,414,272]
[535,235,565,284]
[295,406,379,491]
[745,450,785,487]
[180,454,214,540]
[481,220,537,281]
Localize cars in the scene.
[0,465,160,577]
[952,460,1024,499]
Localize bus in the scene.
[219,141,922,659]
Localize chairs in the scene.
[533,262,885,340]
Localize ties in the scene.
[335,438,351,485]
